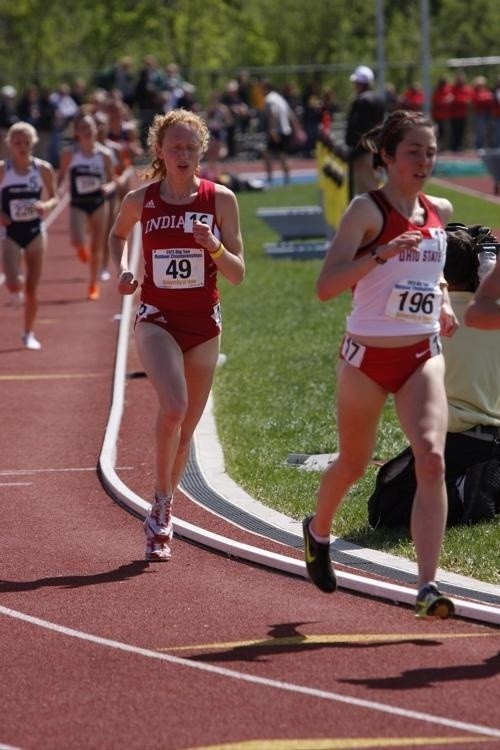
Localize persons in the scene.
[464,247,500,331]
[384,73,500,157]
[57,56,133,301]
[2,53,57,132]
[108,108,245,563]
[206,68,340,161]
[0,120,58,348]
[134,49,203,109]
[300,111,460,620]
[345,65,385,205]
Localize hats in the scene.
[350,65,375,84]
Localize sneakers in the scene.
[99,270,111,282]
[21,332,42,350]
[301,515,338,595]
[75,245,89,263]
[87,284,101,301]
[142,520,173,564]
[413,585,456,623]
[146,491,175,543]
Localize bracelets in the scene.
[209,243,225,260]
[369,248,386,264]
